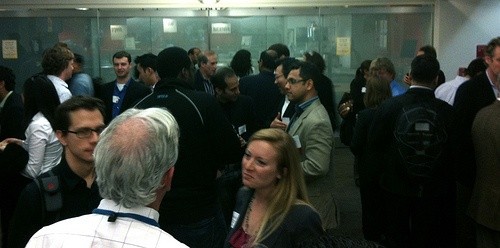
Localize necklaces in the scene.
[245,202,265,241]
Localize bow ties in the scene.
[293,98,318,117]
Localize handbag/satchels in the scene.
[337,92,356,120]
[38,175,63,212]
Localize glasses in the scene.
[274,74,283,80]
[68,124,107,139]
[284,78,305,85]
[258,61,264,64]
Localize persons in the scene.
[224,128,326,248]
[0,43,241,248]
[338,37,500,248]
[216,44,337,142]
[271,62,339,184]
